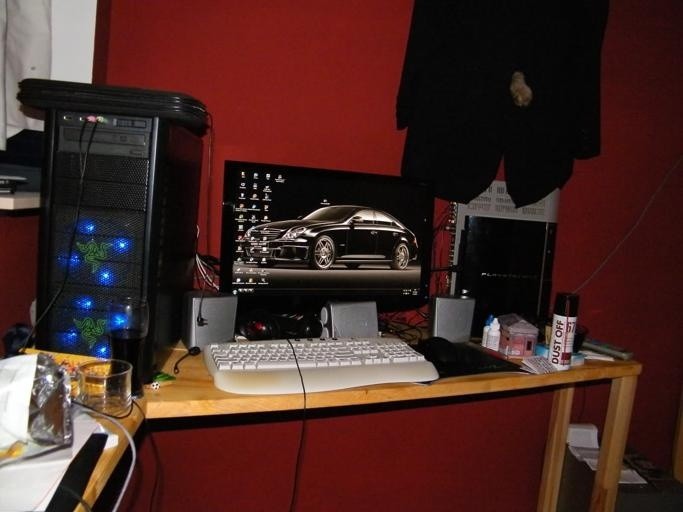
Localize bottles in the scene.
[481,315,500,353]
[547,291,580,372]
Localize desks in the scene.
[2,312,644,512]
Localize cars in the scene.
[244,205,419,270]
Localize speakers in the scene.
[430,293,477,344]
[179,289,239,351]
[320,300,378,339]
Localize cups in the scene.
[107,297,147,400]
[543,321,555,348]
[71,360,132,417]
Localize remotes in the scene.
[582,339,634,360]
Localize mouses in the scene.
[416,336,455,367]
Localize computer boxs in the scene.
[34,109,204,386]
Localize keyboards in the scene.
[203,336,440,395]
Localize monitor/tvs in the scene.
[219,159,437,337]
